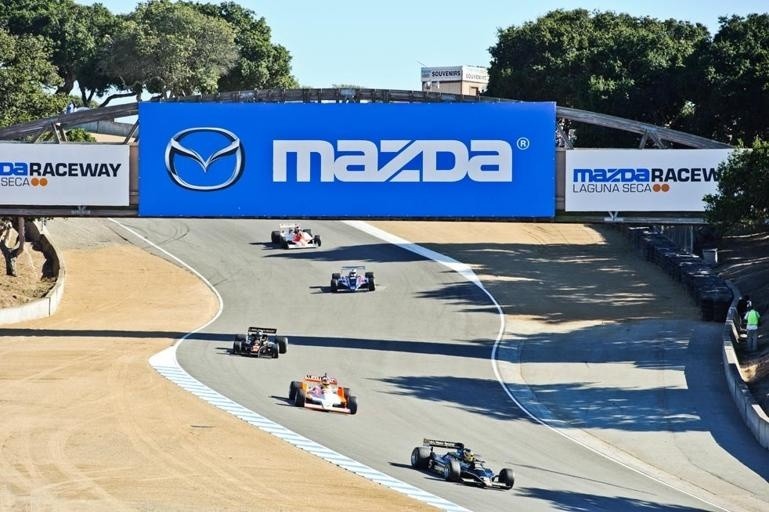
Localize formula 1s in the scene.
[330,265,374,293]
[233,326,287,358]
[288,372,357,415]
[271,224,321,249]
[411,438,514,493]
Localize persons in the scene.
[737,294,753,328]
[459,447,474,464]
[346,268,359,281]
[252,330,266,343]
[293,224,301,234]
[313,375,331,391]
[743,304,760,352]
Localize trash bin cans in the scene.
[702,248,718,268]
